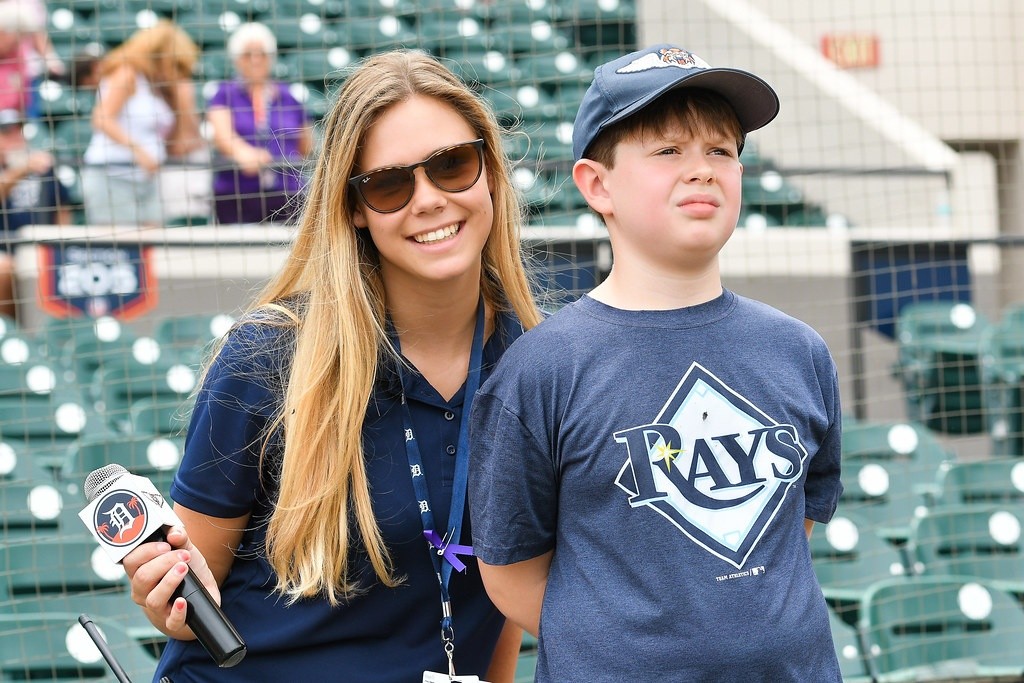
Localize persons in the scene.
[0,0,72,322]
[85,25,200,227]
[206,23,314,224]
[123,52,545,683]
[468,43,845,683]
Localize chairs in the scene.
[0,0,1024,683]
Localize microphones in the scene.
[76,464,246,669]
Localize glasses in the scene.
[346,137,484,214]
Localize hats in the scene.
[572,43,780,162]
